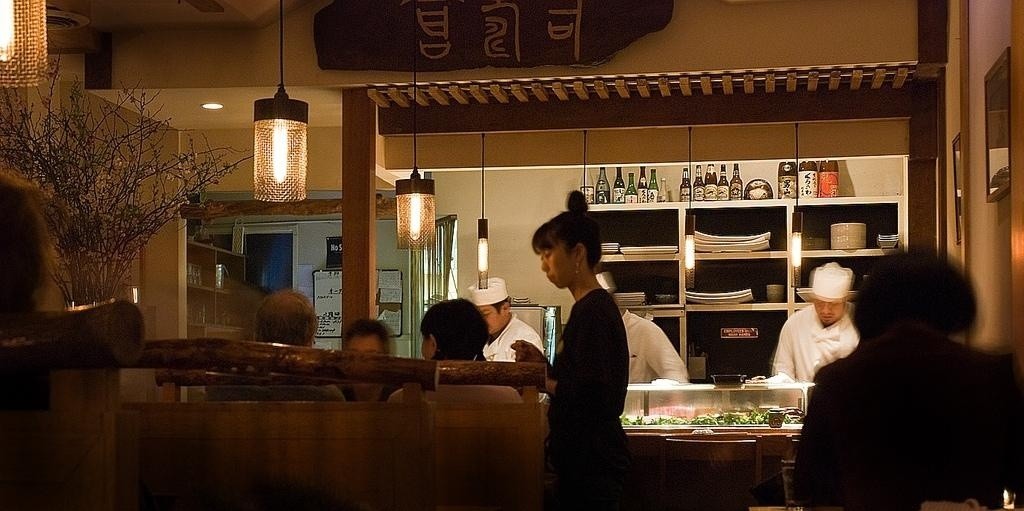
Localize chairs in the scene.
[660,435,762,491]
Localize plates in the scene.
[600,233,774,256]
[611,288,868,307]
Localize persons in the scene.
[765,260,862,408]
[339,317,403,402]
[202,286,348,404]
[465,276,548,405]
[594,269,692,414]
[508,188,633,511]
[387,296,523,405]
[792,244,1024,511]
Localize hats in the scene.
[467,277,508,307]
[596,274,610,291]
[812,267,850,303]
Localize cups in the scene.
[766,410,785,428]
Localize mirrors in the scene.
[951,133,962,244]
[984,48,1011,204]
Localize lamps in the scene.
[791,124,802,267]
[0,0,47,88]
[476,133,489,289]
[396,1,437,252]
[684,127,696,271]
[253,0,308,203]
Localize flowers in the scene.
[0,36,254,306]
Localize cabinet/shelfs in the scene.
[186,241,248,339]
[586,195,908,368]
[620,384,813,459]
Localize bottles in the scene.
[580,161,841,204]
